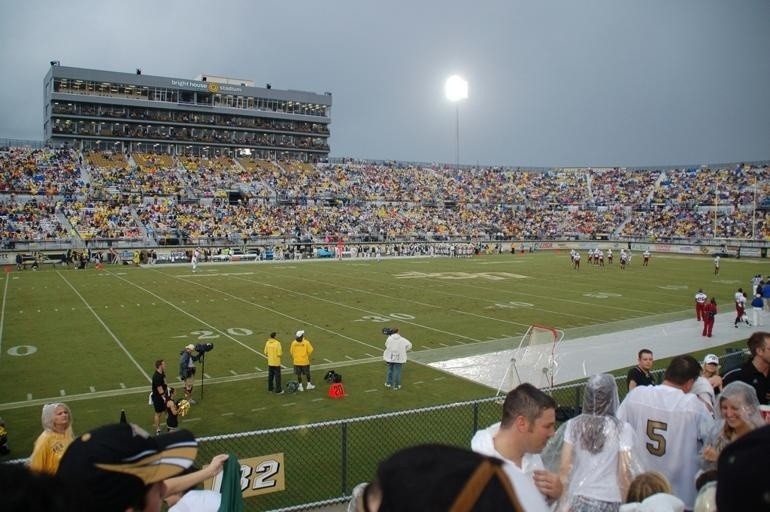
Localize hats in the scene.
[54,423,198,511]
[296,330,304,337]
[358,445,523,512]
[705,355,719,365]
[185,344,194,350]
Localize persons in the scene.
[2,145,769,512]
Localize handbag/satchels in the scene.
[287,382,297,393]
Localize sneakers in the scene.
[307,382,315,390]
[298,383,304,391]
[385,383,401,390]
